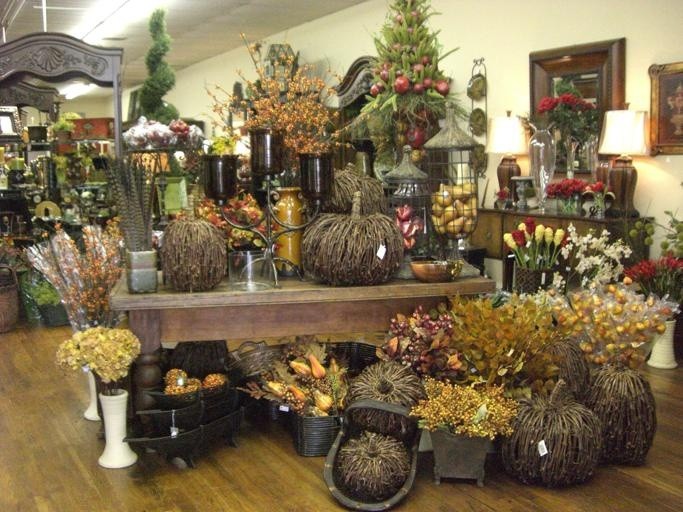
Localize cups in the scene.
[412,259,463,283]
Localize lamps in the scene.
[482,110,529,203]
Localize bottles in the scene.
[0,143,43,186]
[16,215,26,236]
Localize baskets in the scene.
[324,401,422,512]
[228,341,281,377]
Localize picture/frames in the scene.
[647,61,682,156]
[526,36,626,161]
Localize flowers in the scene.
[55,326,143,394]
[243,343,345,415]
[203,30,358,185]
[172,191,276,255]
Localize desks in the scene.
[467,205,655,265]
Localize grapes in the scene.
[122,115,205,149]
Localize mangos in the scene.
[429,181,479,236]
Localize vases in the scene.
[427,427,492,487]
[246,128,285,174]
[646,318,678,369]
[297,150,338,199]
[275,189,304,277]
[224,249,277,293]
[201,155,239,200]
[97,365,244,471]
[81,370,101,422]
[293,411,335,460]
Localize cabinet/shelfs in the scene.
[0,30,126,231]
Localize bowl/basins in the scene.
[226,248,272,293]
